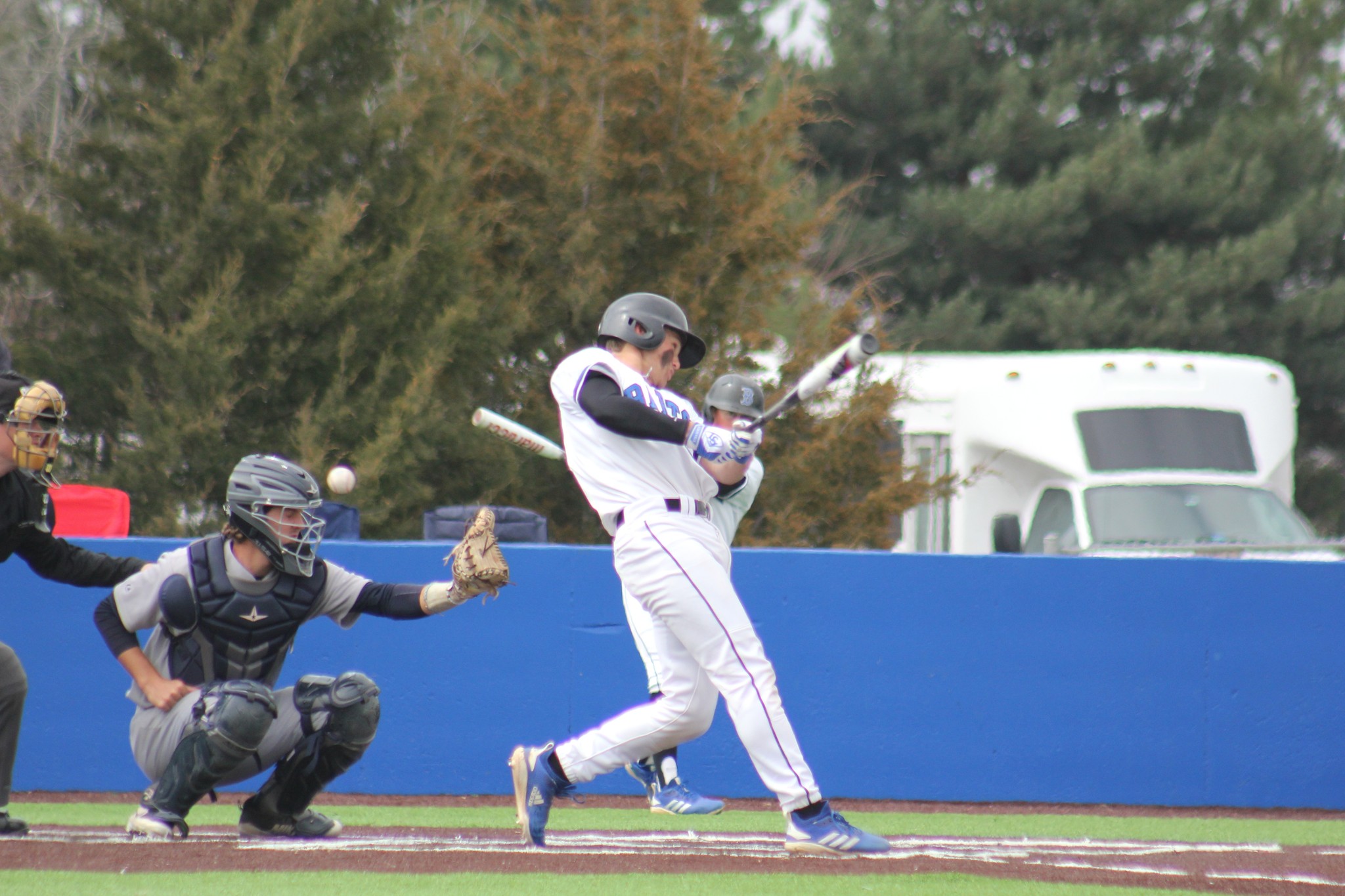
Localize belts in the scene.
[616,499,710,531]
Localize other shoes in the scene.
[0,810,29,837]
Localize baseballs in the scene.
[326,468,356,495]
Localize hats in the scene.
[0,371,57,425]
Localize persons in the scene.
[0,372,152,834]
[483,292,891,857]
[91,455,509,838]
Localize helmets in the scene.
[704,374,763,424]
[597,292,706,369]
[224,454,326,577]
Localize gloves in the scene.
[727,420,762,464]
[685,422,737,464]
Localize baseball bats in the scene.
[745,332,880,434]
[472,407,567,464]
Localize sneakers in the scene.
[508,743,586,851]
[651,775,725,816]
[125,802,189,841]
[623,758,659,800]
[237,794,342,838]
[784,800,891,858]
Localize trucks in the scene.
[810,348,1339,562]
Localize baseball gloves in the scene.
[453,507,511,597]
